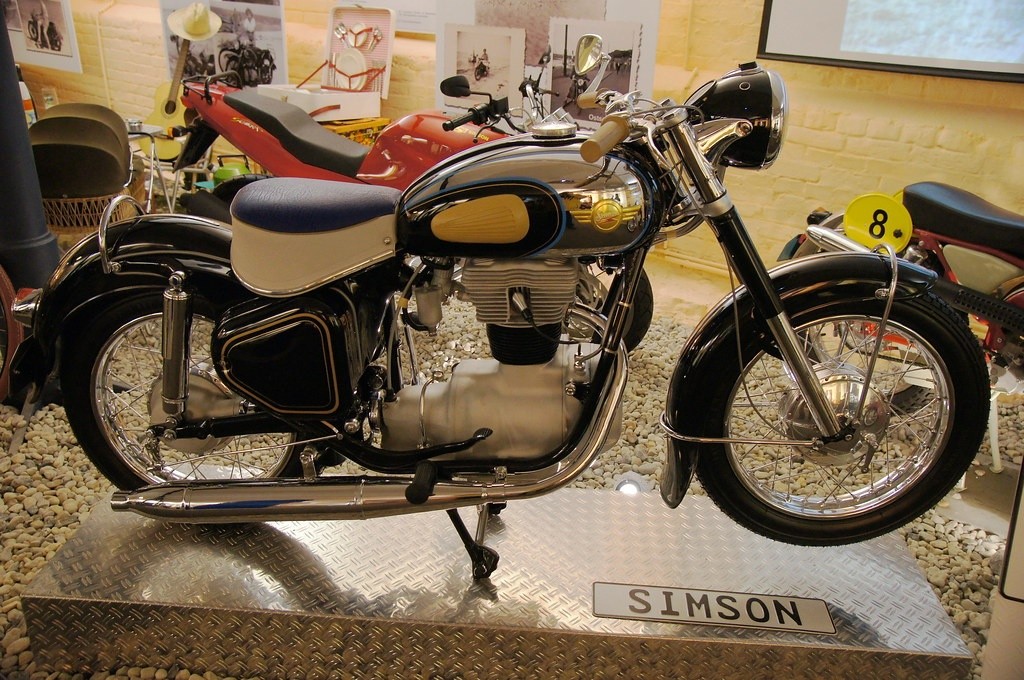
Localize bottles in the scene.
[16,63,38,130]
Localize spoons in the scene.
[338,25,348,38]
[334,27,347,47]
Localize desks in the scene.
[126,124,164,139]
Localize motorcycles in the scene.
[28,19,63,51]
[167,45,656,359]
[762,179,1024,426]
[0,32,992,595]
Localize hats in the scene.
[167,2,222,41]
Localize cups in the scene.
[42,85,61,110]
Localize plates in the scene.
[336,48,367,91]
[167,7,221,42]
[348,21,367,48]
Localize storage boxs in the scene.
[259,6,397,120]
